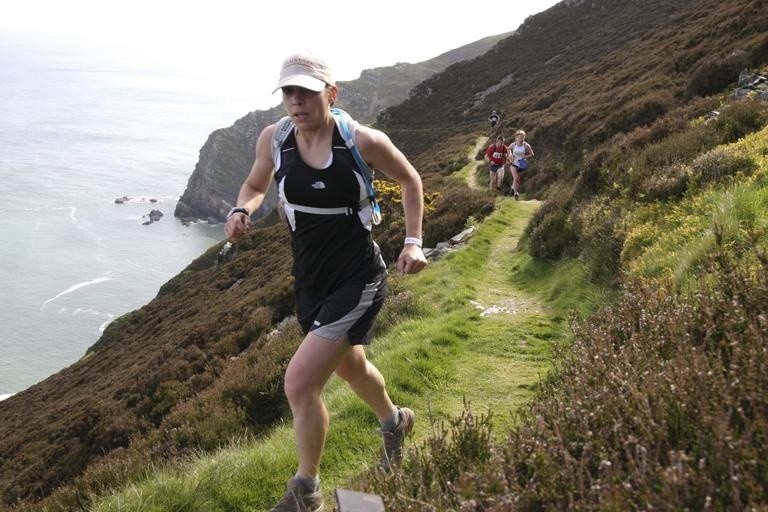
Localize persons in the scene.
[484,135,513,192]
[506,129,535,200]
[488,110,501,128]
[224,51,431,512]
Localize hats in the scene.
[272,56,336,92]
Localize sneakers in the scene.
[510,186,519,200]
[381,406,414,467]
[270,478,324,511]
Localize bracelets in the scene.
[226,205,250,217]
[405,236,423,247]
